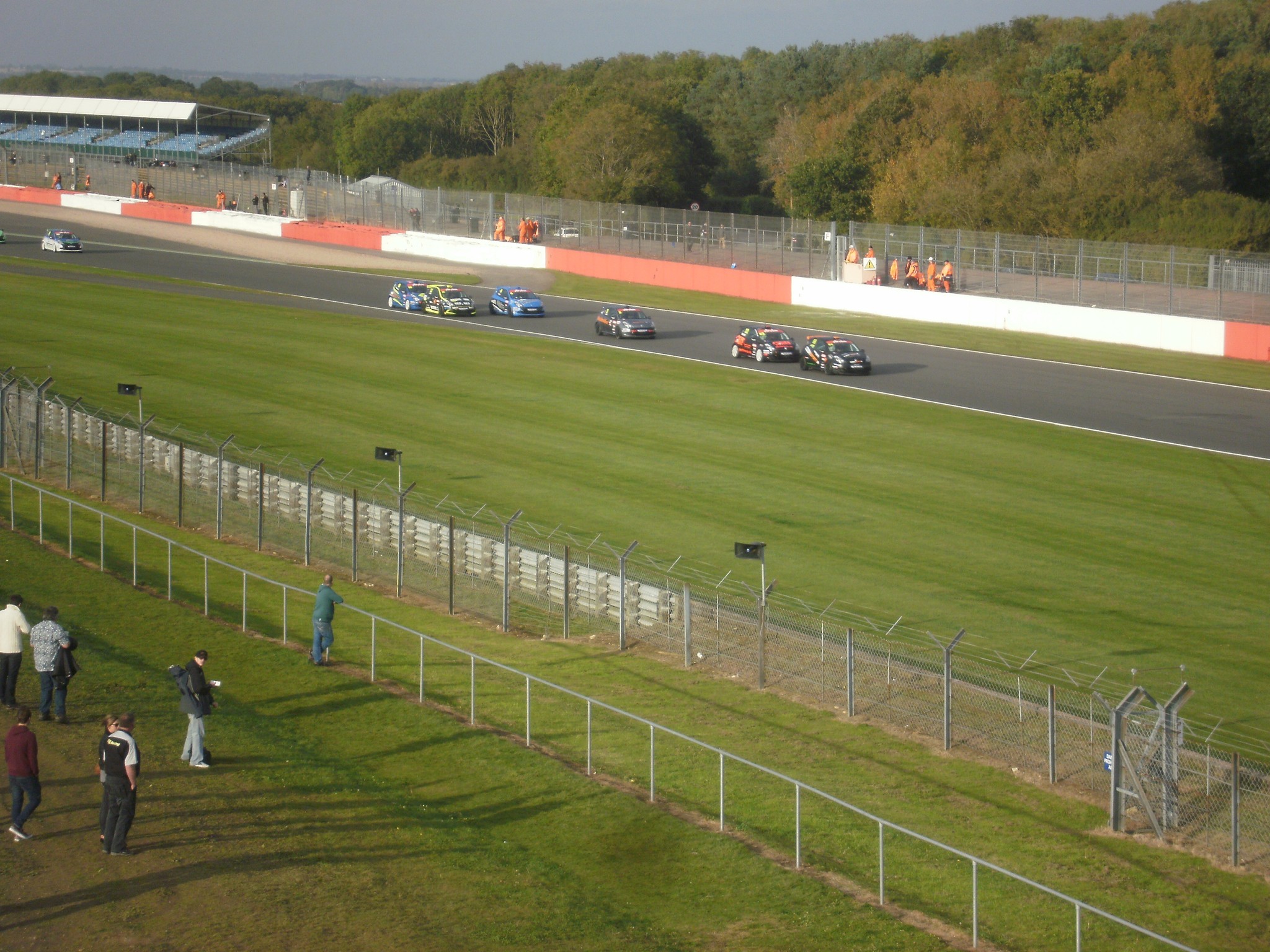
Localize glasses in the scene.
[110,723,119,726]
[198,657,207,661]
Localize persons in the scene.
[518,217,535,244]
[124,152,137,165]
[252,194,259,214]
[904,256,953,292]
[0,594,31,707]
[217,190,225,209]
[5,706,41,841]
[180,650,218,768]
[50,173,62,188]
[865,246,875,258]
[9,152,16,166]
[494,217,505,241]
[131,180,154,199]
[97,711,141,856]
[308,575,343,666]
[410,208,421,231]
[686,222,726,251]
[30,606,69,724]
[845,245,858,263]
[262,193,268,215]
[306,166,310,185]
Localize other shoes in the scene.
[9,824,28,840]
[38,713,50,721]
[190,762,210,768]
[14,833,32,842]
[102,849,110,853]
[5,704,25,710]
[110,849,130,856]
[314,661,324,665]
[308,650,314,662]
[54,714,69,723]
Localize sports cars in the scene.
[420,284,477,316]
[800,334,873,375]
[488,285,546,318]
[731,323,800,363]
[0,228,7,244]
[594,306,657,340]
[41,229,83,253]
[388,280,427,311]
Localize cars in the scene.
[554,228,579,238]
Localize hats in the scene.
[849,245,854,248]
[195,650,208,658]
[927,257,933,261]
